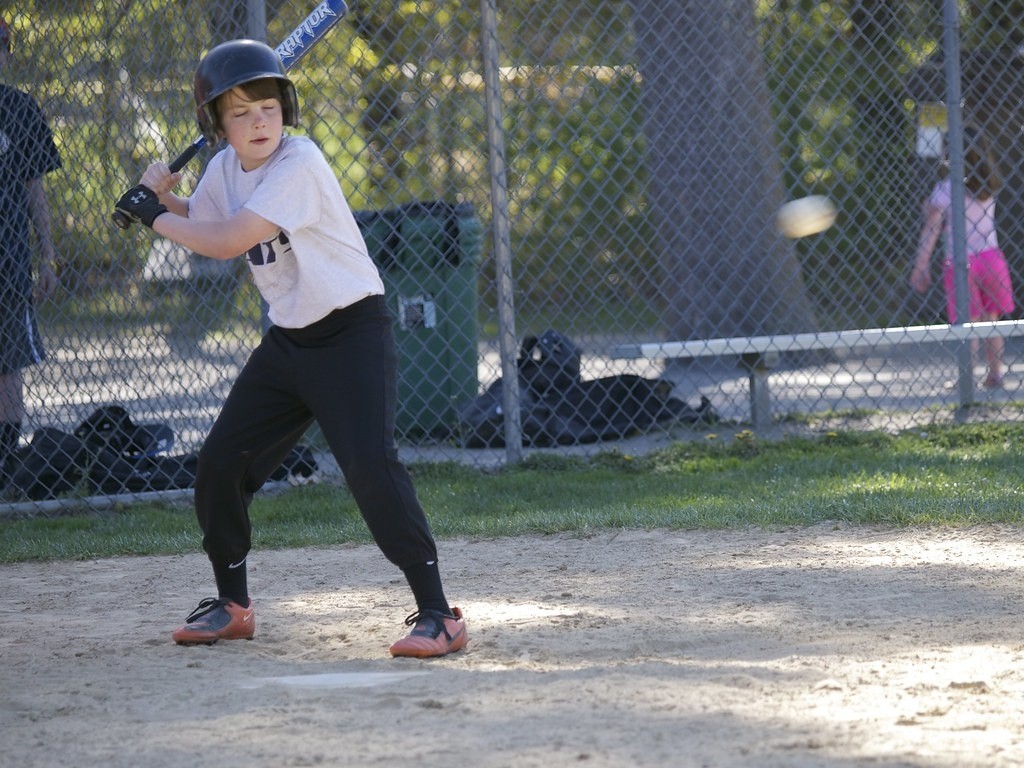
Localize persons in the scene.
[115,39,470,658]
[0,17,62,460]
[910,123,1016,421]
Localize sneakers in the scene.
[172,597,254,646]
[390,607,467,658]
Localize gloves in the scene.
[114,183,169,229]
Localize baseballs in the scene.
[777,191,840,242]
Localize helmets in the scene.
[193,39,299,150]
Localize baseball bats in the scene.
[109,0,349,230]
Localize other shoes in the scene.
[982,371,1003,388]
[943,376,977,390]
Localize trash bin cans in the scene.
[296,199,481,453]
